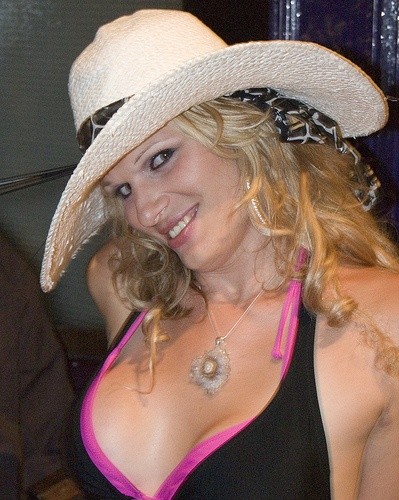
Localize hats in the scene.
[40,8,389,291]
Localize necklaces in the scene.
[187,259,287,397]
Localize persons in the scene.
[0,232,81,500]
[36,7,399,500]
